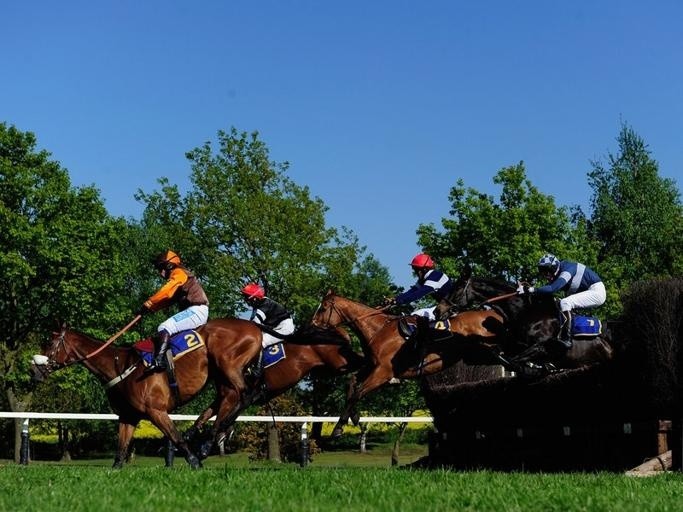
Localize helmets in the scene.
[242,285,263,299]
[537,255,561,276]
[409,255,432,268]
[157,251,180,278]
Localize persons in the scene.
[515,253,607,351]
[240,283,296,380]
[138,248,210,372]
[383,254,459,377]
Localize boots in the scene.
[143,329,170,374]
[251,348,264,376]
[551,311,573,348]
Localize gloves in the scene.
[516,285,534,295]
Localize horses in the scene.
[25,315,351,472]
[298,288,506,442]
[179,315,359,459]
[431,262,612,376]
